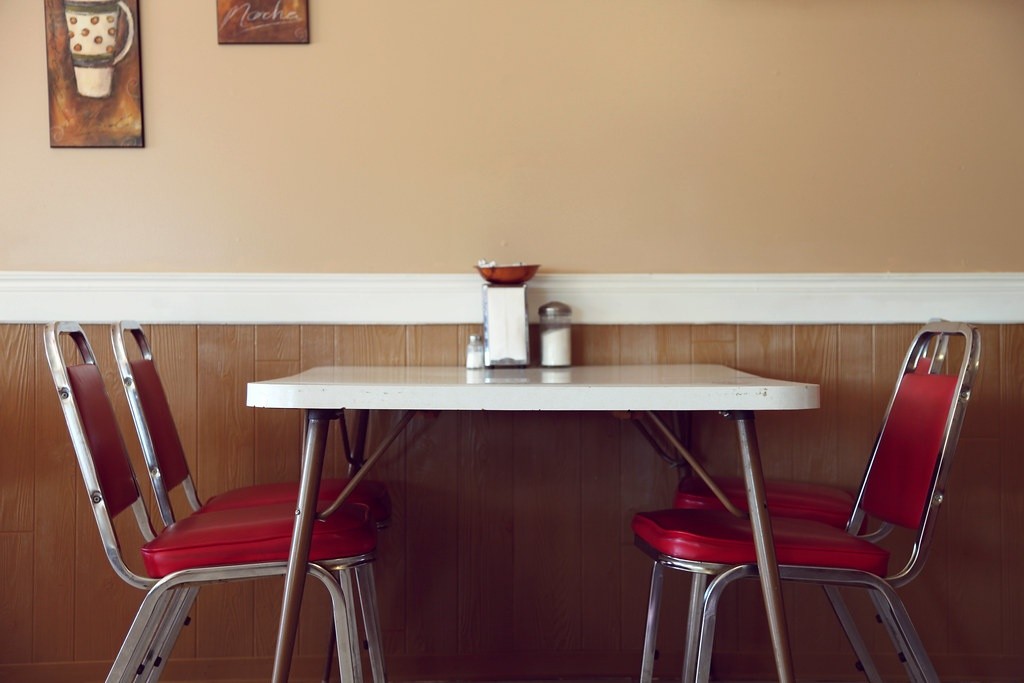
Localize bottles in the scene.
[465,335,483,369]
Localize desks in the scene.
[247,364,822,683]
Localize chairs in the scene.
[631,321,981,682]
[43,319,393,683]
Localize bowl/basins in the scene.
[474,263,541,284]
